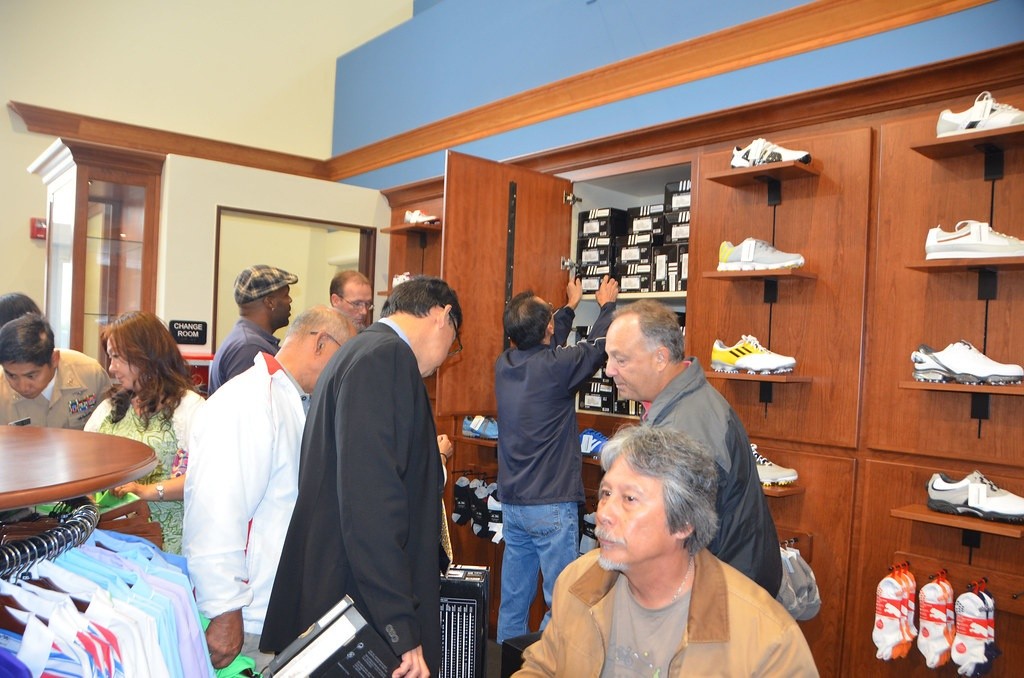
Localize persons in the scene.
[208,265,298,398]
[259,273,462,678]
[0,292,113,431]
[179,306,358,673]
[330,270,372,335]
[605,299,784,599]
[510,425,820,678]
[494,274,617,646]
[82,309,205,555]
[434,434,453,485]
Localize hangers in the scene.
[0,498,164,639]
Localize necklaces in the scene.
[673,554,694,601]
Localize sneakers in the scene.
[925,220,1024,260]
[392,272,412,288]
[925,470,1024,525]
[404,210,440,225]
[731,139,811,168]
[911,340,1024,386]
[462,416,499,439]
[751,443,799,486]
[711,334,796,374]
[936,92,1024,138]
[579,429,612,460]
[716,238,805,271]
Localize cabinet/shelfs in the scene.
[704,121,1024,541]
[435,148,692,420]
[26,136,167,388]
[448,433,807,496]
[376,224,442,295]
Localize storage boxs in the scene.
[577,311,685,415]
[575,179,692,295]
[267,594,410,678]
[441,564,492,678]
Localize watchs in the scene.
[156,482,164,502]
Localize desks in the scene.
[0,422,159,509]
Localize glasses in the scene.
[341,296,375,311]
[441,302,463,356]
[548,302,554,317]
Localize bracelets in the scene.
[440,453,448,464]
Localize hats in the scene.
[233,265,298,306]
